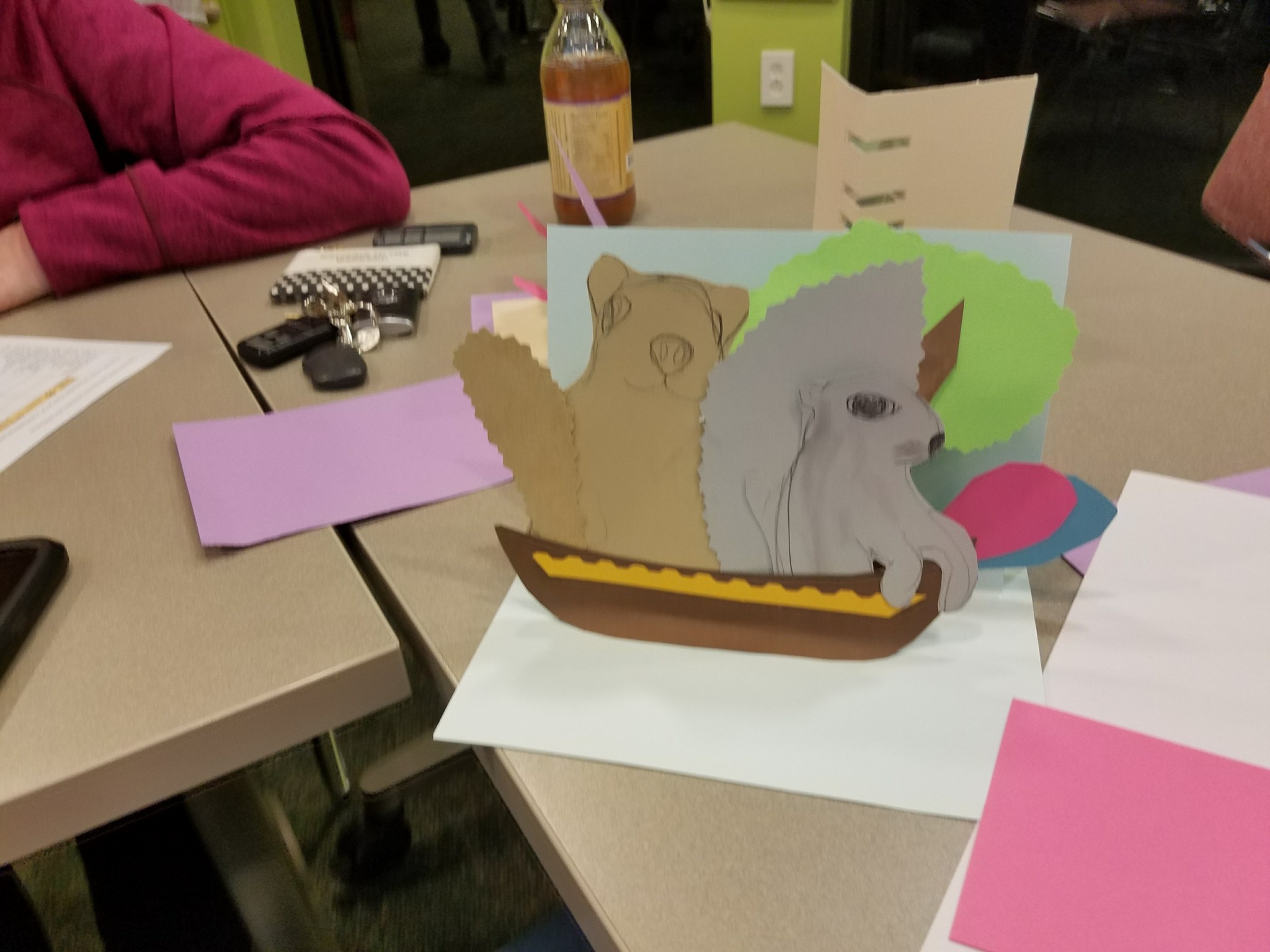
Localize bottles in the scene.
[539,0,636,228]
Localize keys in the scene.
[231,275,414,389]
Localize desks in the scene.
[0,123,1270,951]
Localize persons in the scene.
[1,1,414,331]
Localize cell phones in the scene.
[0,537,69,681]
[373,223,480,253]
